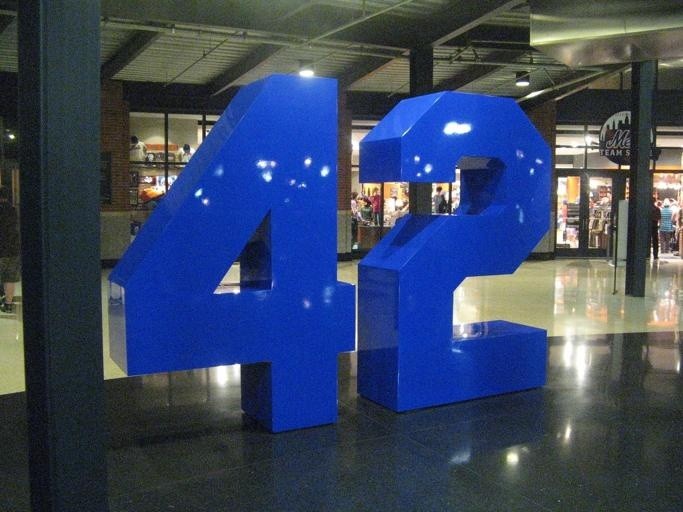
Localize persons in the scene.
[646,259,658,281]
[130,135,147,162]
[646,196,661,260]
[174,144,197,164]
[383,185,461,228]
[350,191,369,249]
[557,198,567,241]
[0,184,22,314]
[368,187,380,226]
[653,198,682,256]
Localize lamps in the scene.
[515,71,530,86]
[298,59,314,75]
